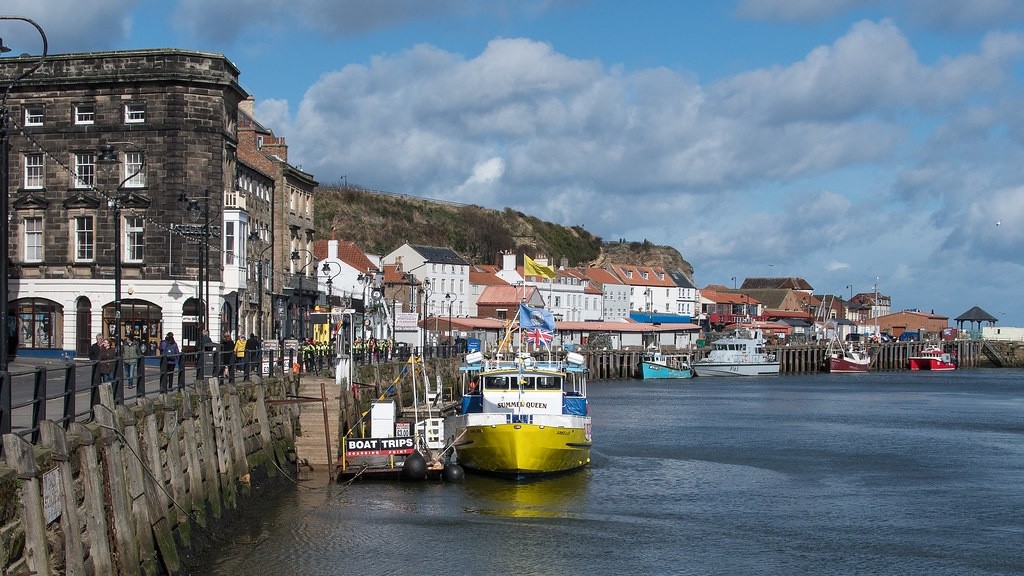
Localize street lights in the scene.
[178,187,220,378]
[288,249,314,340]
[846,284,852,303]
[871,275,879,336]
[247,228,275,350]
[358,272,376,366]
[418,277,433,357]
[388,275,402,358]
[444,291,457,357]
[643,289,653,323]
[401,273,414,313]
[94,141,145,356]
[321,260,341,368]
[739,294,750,305]
[731,277,736,289]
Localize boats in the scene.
[689,322,781,377]
[447,258,591,478]
[905,345,957,372]
[641,352,694,380]
[823,321,870,373]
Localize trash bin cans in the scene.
[696,340,706,348]
[305,351,314,371]
[398,342,407,351]
[202,343,224,385]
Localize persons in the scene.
[90,332,396,391]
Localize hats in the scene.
[126,334,134,342]
[308,338,313,342]
[167,332,173,338]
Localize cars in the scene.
[844,329,919,344]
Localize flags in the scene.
[522,329,553,346]
[520,303,554,329]
[523,255,556,280]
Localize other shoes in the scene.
[169,388,173,391]
[159,391,164,394]
[128,384,133,389]
